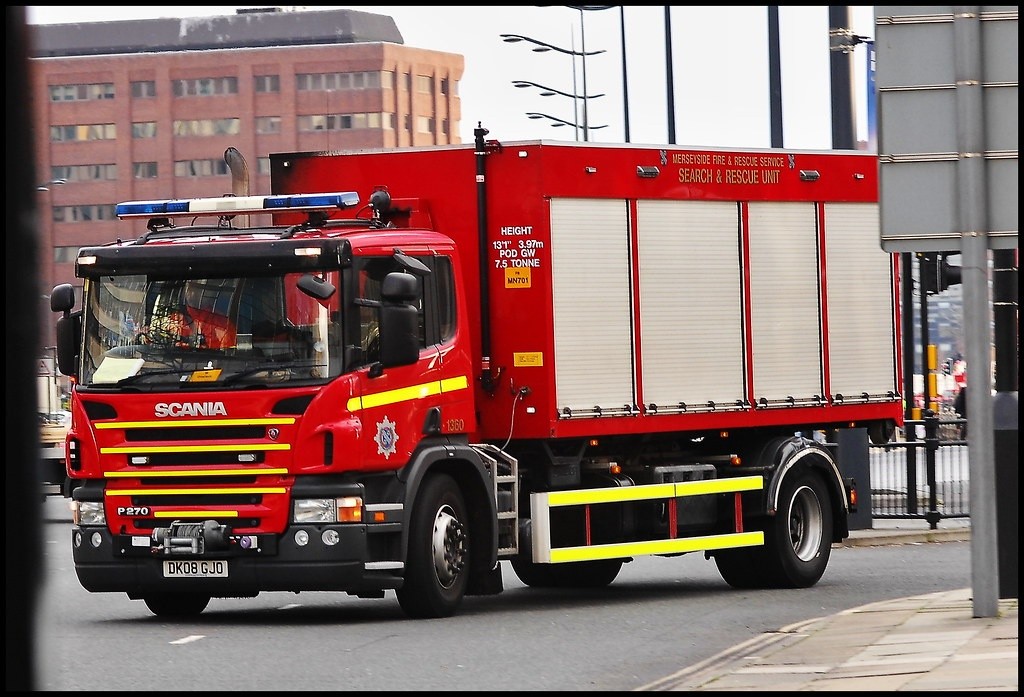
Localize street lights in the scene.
[512,80,604,142]
[526,113,606,141]
[500,34,605,142]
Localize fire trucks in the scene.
[50,117,905,620]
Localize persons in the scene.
[956,388,967,441]
[134,278,237,350]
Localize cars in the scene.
[39,410,72,441]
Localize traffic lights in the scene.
[921,254,964,295]
[942,359,952,375]
